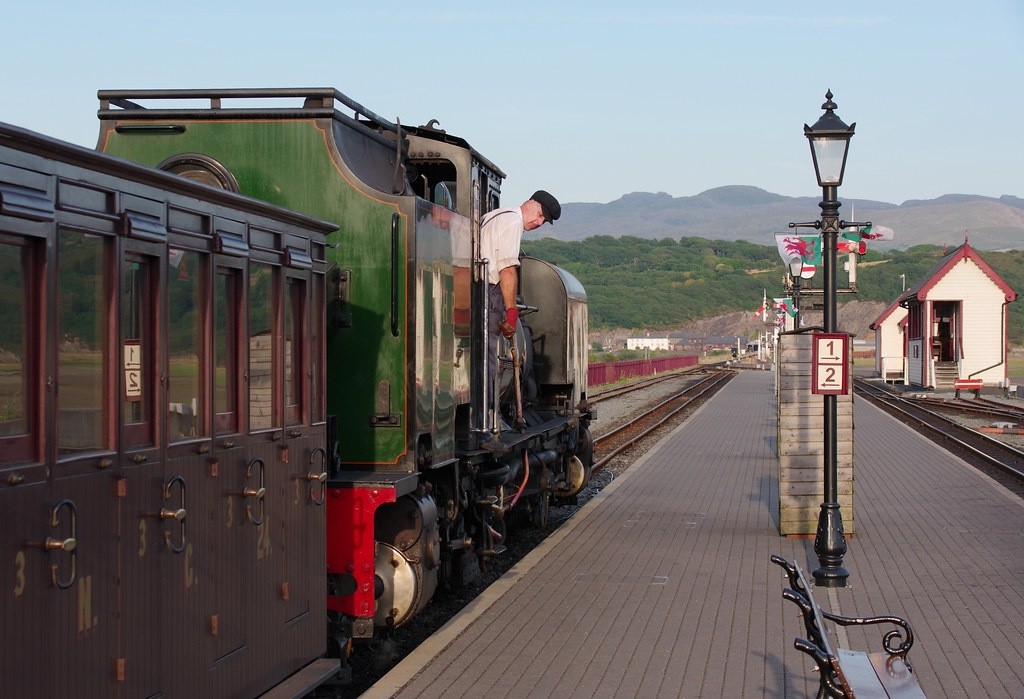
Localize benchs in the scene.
[770,554,927,699]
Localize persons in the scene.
[474,190,561,452]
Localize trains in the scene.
[0,86,603,699]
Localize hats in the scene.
[530,190,561,225]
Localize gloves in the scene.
[498,308,519,339]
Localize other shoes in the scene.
[479,438,512,453]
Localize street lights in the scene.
[789,87,873,588]
